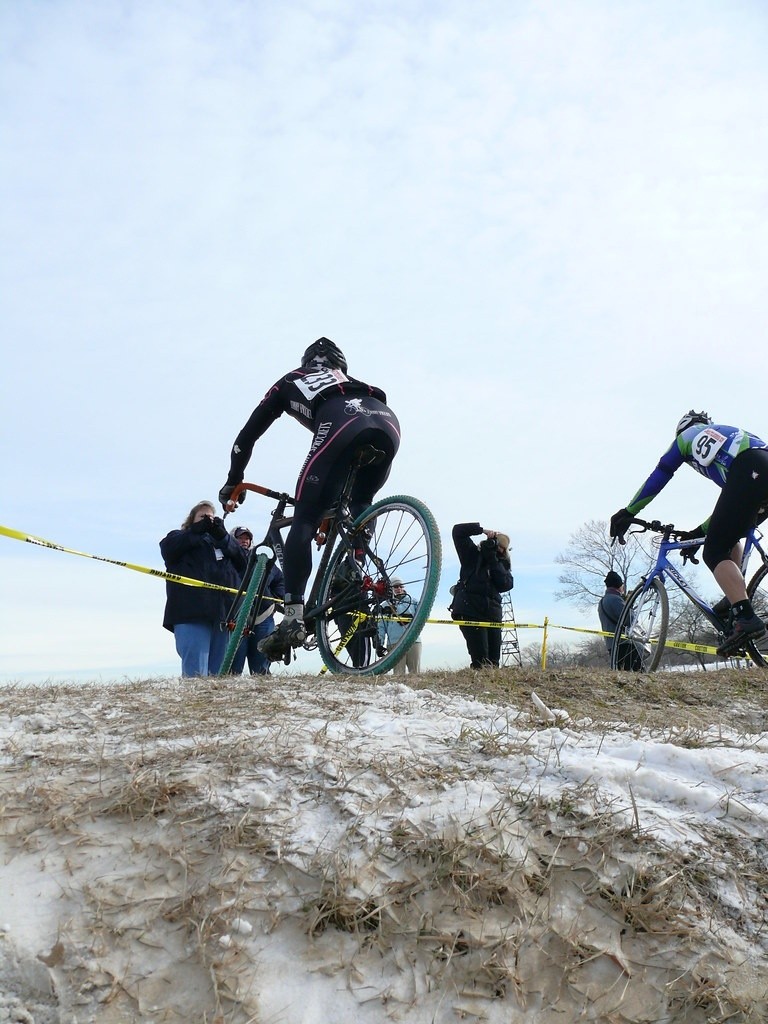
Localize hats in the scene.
[604,571,622,587]
[494,531,510,557]
[235,528,253,540]
[390,577,403,585]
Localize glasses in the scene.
[394,585,404,589]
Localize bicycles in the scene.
[216,442,442,681]
[610,517,768,677]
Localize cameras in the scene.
[212,517,223,526]
[486,537,498,551]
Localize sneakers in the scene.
[716,612,766,655]
[257,618,306,653]
[332,558,361,583]
[712,596,732,618]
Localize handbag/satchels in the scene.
[630,623,651,661]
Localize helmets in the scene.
[301,336,347,375]
[675,410,713,436]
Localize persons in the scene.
[377,576,424,674]
[159,502,248,677]
[219,336,401,650]
[610,412,768,655]
[222,527,286,676]
[598,571,645,672]
[450,522,513,669]
[330,546,378,668]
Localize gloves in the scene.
[610,508,634,536]
[377,647,386,657]
[219,473,247,514]
[680,524,705,556]
